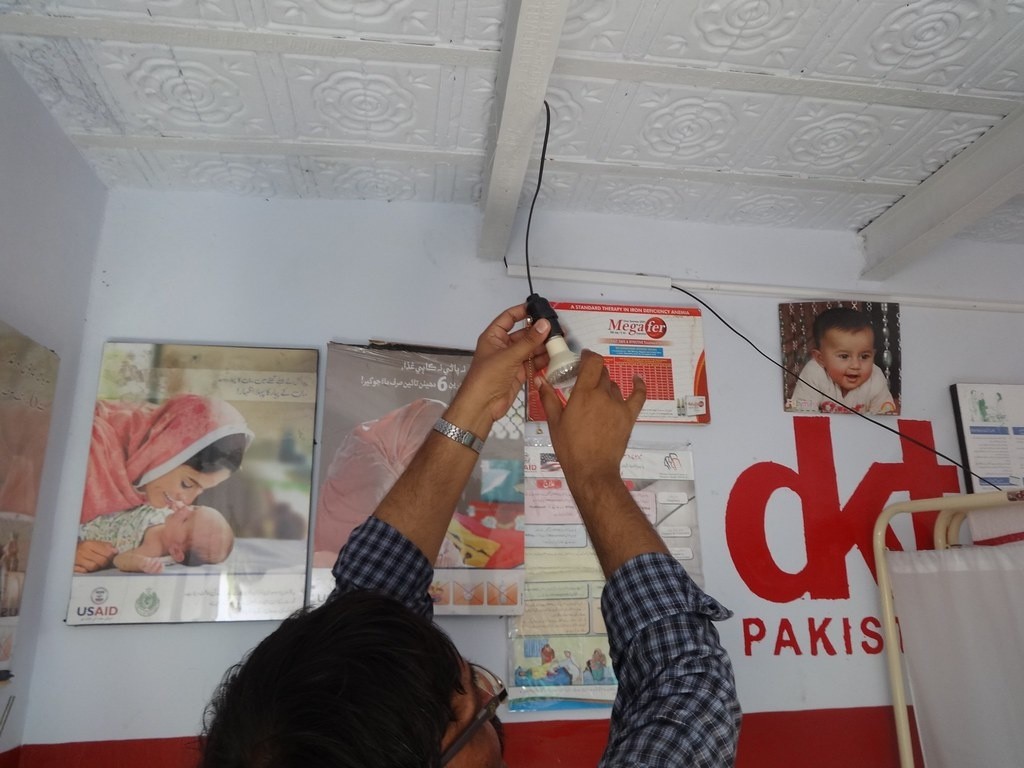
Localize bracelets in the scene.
[433,416,485,454]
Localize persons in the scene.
[196,298,748,767]
[963,388,1008,426]
[76,497,237,573]
[73,391,253,576]
[787,307,895,413]
[311,396,468,567]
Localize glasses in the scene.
[437,662,508,767]
